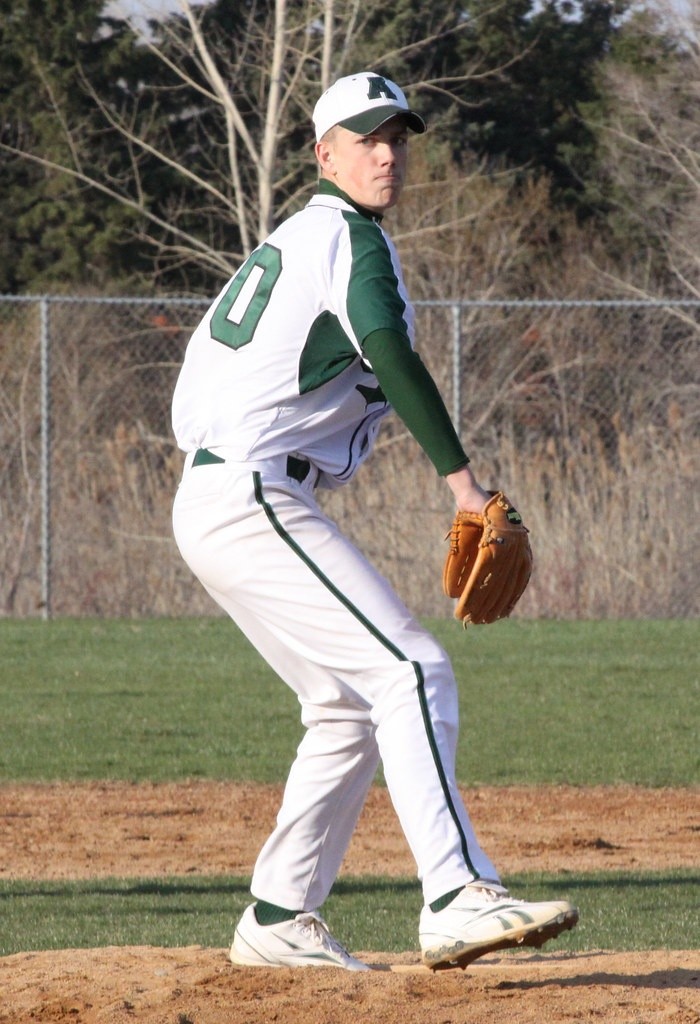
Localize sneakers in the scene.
[229,902,371,973]
[419,879,578,972]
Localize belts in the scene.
[191,449,321,490]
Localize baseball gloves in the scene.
[440,488,535,632]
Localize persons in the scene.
[171,71,576,971]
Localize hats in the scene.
[312,71,427,142]
